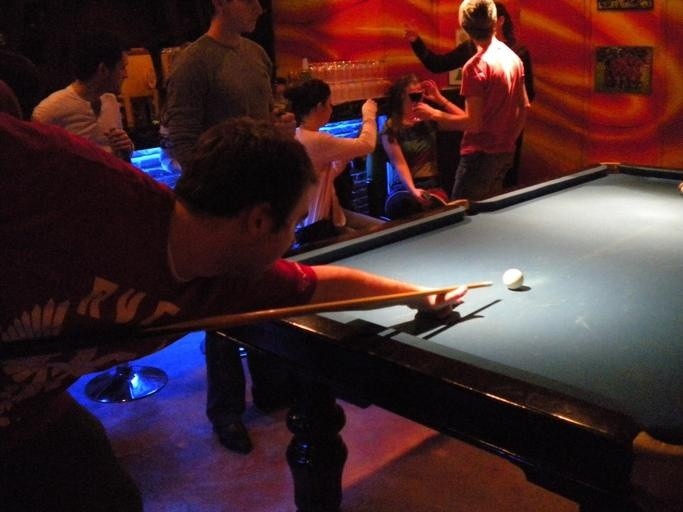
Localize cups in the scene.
[308,61,385,85]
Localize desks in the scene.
[206,160,682,512]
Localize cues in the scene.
[1,283,493,361]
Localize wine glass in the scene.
[408,86,425,122]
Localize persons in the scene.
[378,75,466,210]
[160,1,296,173]
[405,0,535,200]
[31,32,134,155]
[1,110,467,512]
[277,76,378,250]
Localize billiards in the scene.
[504,268,522,290]
